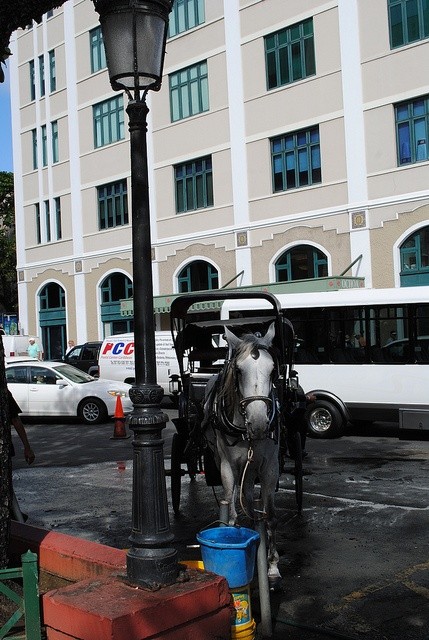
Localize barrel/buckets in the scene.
[221,585,256,640]
[196,520,260,588]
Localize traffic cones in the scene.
[111,394,129,440]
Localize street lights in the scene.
[91,2,179,587]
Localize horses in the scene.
[203,321,284,592]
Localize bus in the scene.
[218,287,429,439]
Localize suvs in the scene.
[55,341,99,378]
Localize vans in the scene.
[99,331,218,404]
[1,335,45,360]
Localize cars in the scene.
[4,362,134,424]
[3,357,39,364]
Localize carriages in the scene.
[164,289,309,524]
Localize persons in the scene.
[65,340,77,358]
[27,338,40,360]
[8,391,35,522]
